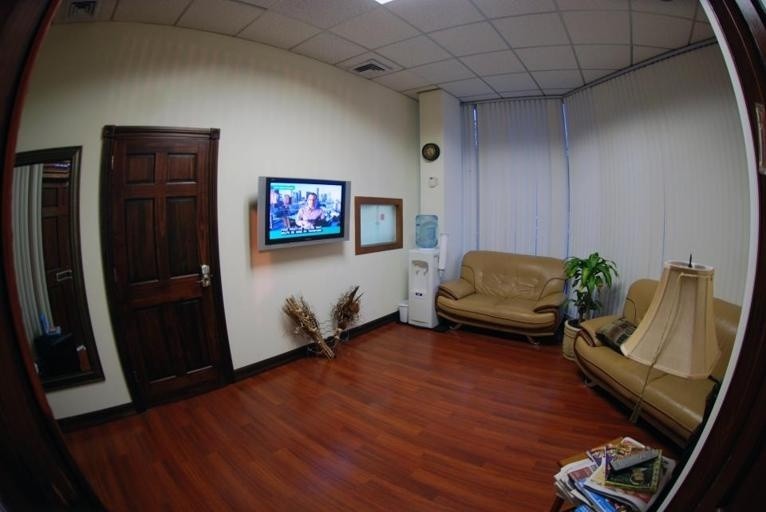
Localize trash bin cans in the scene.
[399,300,408,323]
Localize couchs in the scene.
[573,277,742,455]
[435,250,572,347]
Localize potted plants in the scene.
[556,251,621,361]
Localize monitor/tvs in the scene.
[257,175,351,253]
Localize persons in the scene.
[270,188,330,230]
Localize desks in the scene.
[548,437,626,512]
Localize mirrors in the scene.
[12,144,105,396]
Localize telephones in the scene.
[47,326,62,337]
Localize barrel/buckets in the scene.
[415,214,438,248]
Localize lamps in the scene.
[618,253,726,386]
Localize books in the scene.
[553,436,677,512]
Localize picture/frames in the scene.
[354,195,406,253]
